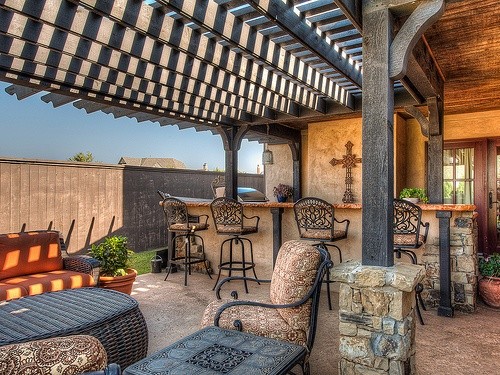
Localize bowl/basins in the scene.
[402,197,419,204]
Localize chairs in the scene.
[201,240,334,375]
[0,335,121,375]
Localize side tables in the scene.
[120,326,307,375]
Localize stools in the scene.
[210,197,261,293]
[293,197,350,310]
[391,198,430,325]
[162,197,212,286]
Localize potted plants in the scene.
[87,236,138,296]
[399,188,429,205]
[478,252,500,309]
[273,183,295,203]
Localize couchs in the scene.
[0,229,100,302]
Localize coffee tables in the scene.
[0,287,148,370]
